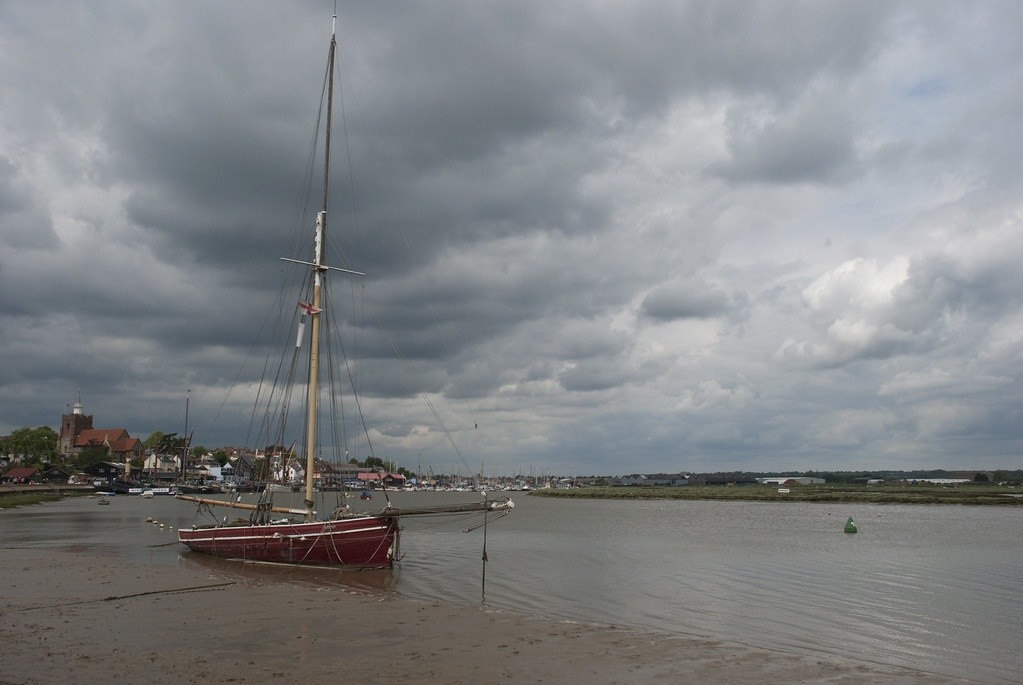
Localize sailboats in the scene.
[264,439,298,493]
[315,463,579,494]
[173,18,514,573]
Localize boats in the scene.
[168,491,183,496]
[166,478,268,493]
[143,490,154,499]
[298,486,319,493]
[98,500,111,505]
